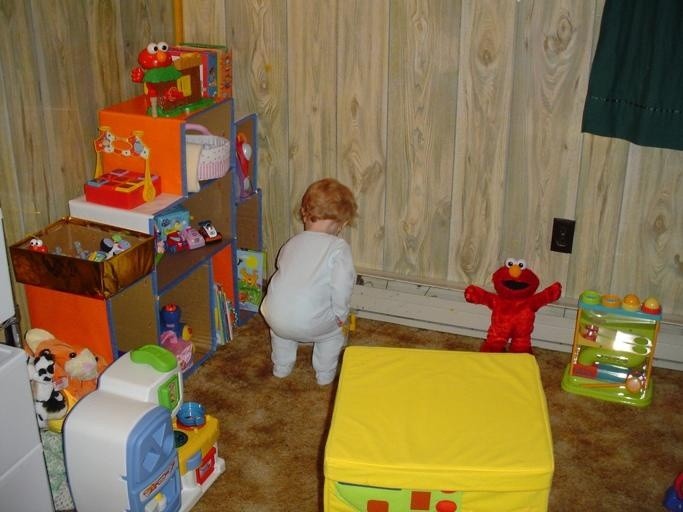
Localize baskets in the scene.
[175,122,232,181]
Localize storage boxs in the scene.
[321,343,553,511]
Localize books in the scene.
[170,43,233,101]
[213,277,241,342]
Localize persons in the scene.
[257,176,358,385]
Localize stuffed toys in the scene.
[27,349,67,428]
[461,257,562,354]
[25,328,103,397]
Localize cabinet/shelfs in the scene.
[0,344,54,512]
[9,93,269,383]
